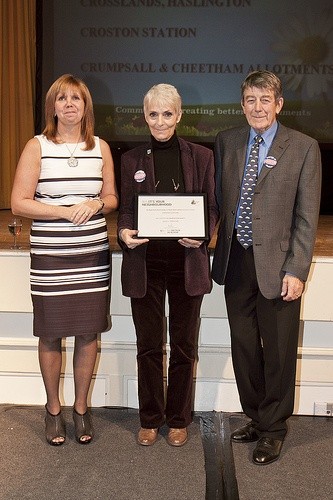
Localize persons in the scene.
[211,71,322,464]
[116,84,221,446]
[11,75,118,445]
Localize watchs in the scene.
[93,198,105,214]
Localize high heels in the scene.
[44,401,66,446]
[72,402,93,444]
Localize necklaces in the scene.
[57,130,81,166]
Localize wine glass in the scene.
[8,218,23,250]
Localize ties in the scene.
[236,134,263,250]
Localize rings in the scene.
[295,293,300,297]
[83,213,88,218]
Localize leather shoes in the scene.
[230,421,262,443]
[252,436,283,465]
[166,427,188,446]
[137,427,158,445]
